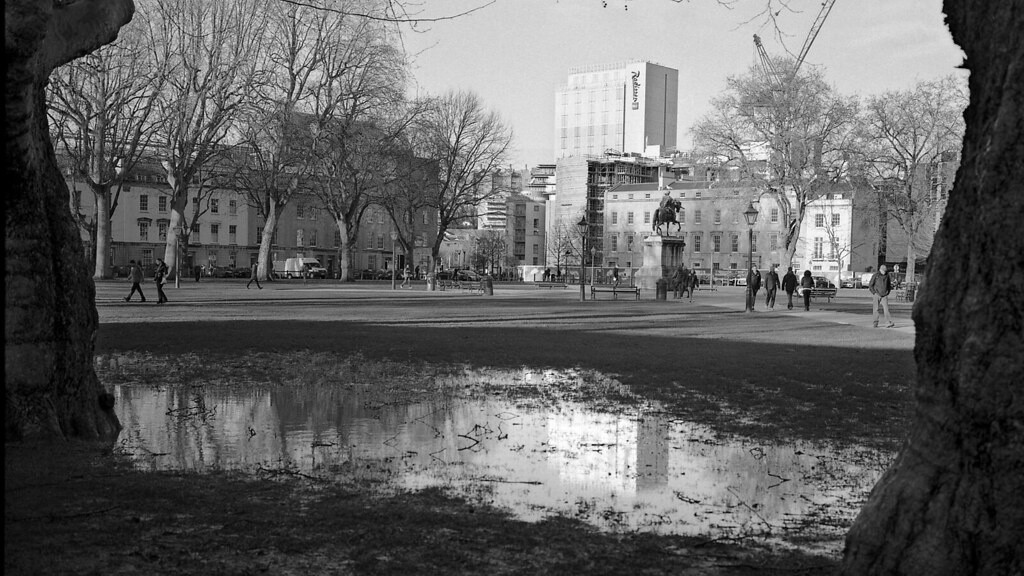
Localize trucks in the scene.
[285,258,327,278]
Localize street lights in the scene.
[566,249,570,284]
[173,222,183,289]
[390,227,398,289]
[576,215,590,302]
[743,199,759,310]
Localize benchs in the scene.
[591,286,640,300]
[792,289,836,303]
[539,283,567,290]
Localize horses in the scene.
[652,200,682,236]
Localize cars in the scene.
[352,268,404,280]
[206,267,251,278]
[843,278,862,288]
[696,274,747,285]
[437,270,481,281]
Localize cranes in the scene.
[753,0,835,104]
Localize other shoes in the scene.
[886,321,894,327]
[124,296,130,302]
[140,298,145,302]
[765,303,768,309]
[769,307,773,311]
[873,321,878,328]
[157,298,167,304]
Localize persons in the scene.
[400,263,412,289]
[746,265,761,310]
[782,266,798,310]
[153,258,168,304]
[247,262,264,289]
[659,191,676,218]
[764,266,780,310]
[465,265,470,270]
[509,269,514,280]
[194,263,214,282]
[327,263,332,277]
[415,265,420,280]
[672,265,700,305]
[301,263,309,278]
[801,270,816,312]
[869,265,895,328]
[451,267,460,280]
[612,264,619,290]
[124,258,147,302]
